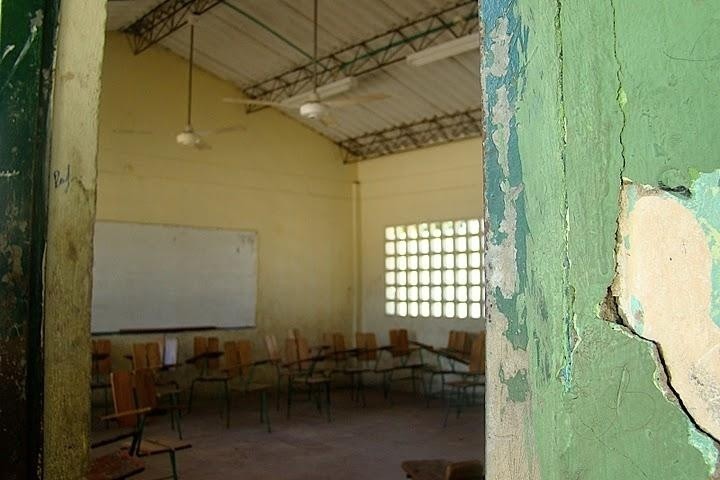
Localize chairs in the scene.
[111,370,191,480]
[90,329,485,437]
[90,405,155,480]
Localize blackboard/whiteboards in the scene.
[90,219,258,334]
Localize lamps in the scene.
[405,34,479,69]
[282,77,359,111]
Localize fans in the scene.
[220,3,391,131]
[111,2,247,152]
